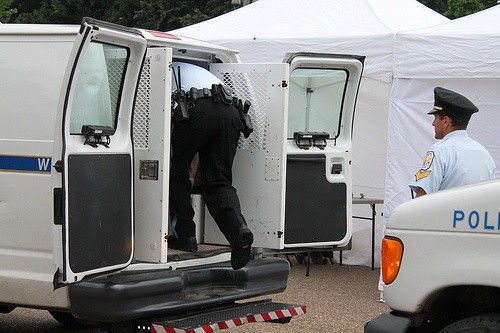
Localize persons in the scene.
[408,87,496,197]
[168,61,255,271]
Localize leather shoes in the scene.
[168,235,198,252]
[228,224,253,270]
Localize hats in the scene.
[427,87,479,114]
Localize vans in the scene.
[0,15,367,333]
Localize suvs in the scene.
[364,176,500,333]
